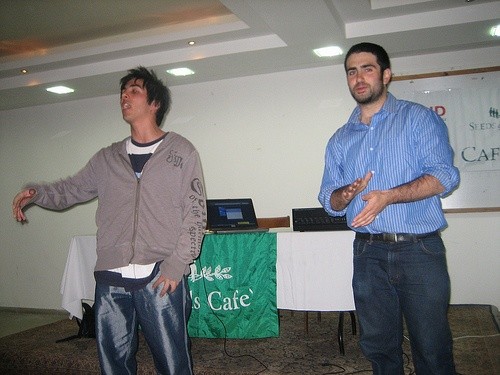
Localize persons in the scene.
[317,41,461,375]
[12,68,208,375]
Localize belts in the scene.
[355,231,438,242]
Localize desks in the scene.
[55,226,356,357]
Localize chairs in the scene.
[252,216,322,336]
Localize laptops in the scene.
[206,198,269,234]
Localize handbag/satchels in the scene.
[75,303,96,338]
